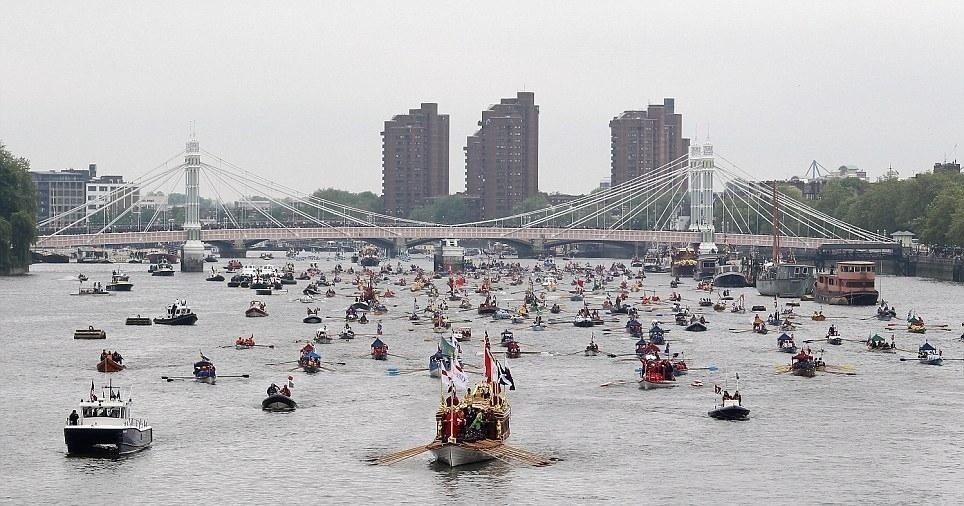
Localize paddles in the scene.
[216,375,249,378]
[161,376,195,381]
[321,362,346,365]
[327,287,945,361]
[688,367,719,371]
[254,345,274,348]
[460,440,563,468]
[367,441,442,465]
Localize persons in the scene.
[69,248,942,443]
